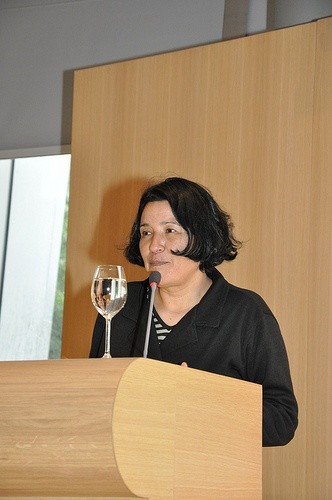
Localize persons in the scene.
[88,178,301,449]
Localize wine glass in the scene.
[90,265,128,358]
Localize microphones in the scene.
[143,271,161,358]
